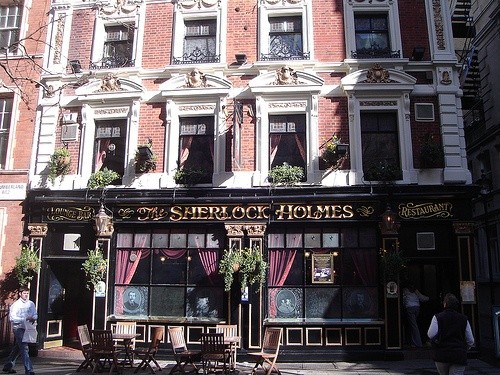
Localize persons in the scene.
[402,280,429,348]
[101,74,121,92]
[428,293,474,375]
[279,65,295,85]
[440,71,452,85]
[3,286,38,375]
[124,291,139,310]
[189,69,204,88]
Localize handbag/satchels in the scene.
[424,332,442,348]
[21,319,37,343]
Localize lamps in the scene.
[235,54,246,62]
[412,47,426,59]
[70,60,81,69]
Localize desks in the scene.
[197,336,241,375]
[112,334,142,373]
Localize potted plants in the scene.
[12,245,40,285]
[136,143,153,160]
[48,149,71,181]
[81,248,108,290]
[322,134,349,154]
[218,250,268,292]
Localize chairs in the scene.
[200,333,229,375]
[216,324,237,367]
[90,329,121,375]
[132,327,163,374]
[77,325,98,371]
[116,322,136,365]
[247,327,283,375]
[169,327,200,375]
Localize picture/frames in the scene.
[312,254,334,284]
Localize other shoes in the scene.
[2,367,16,373]
[25,371,35,375]
